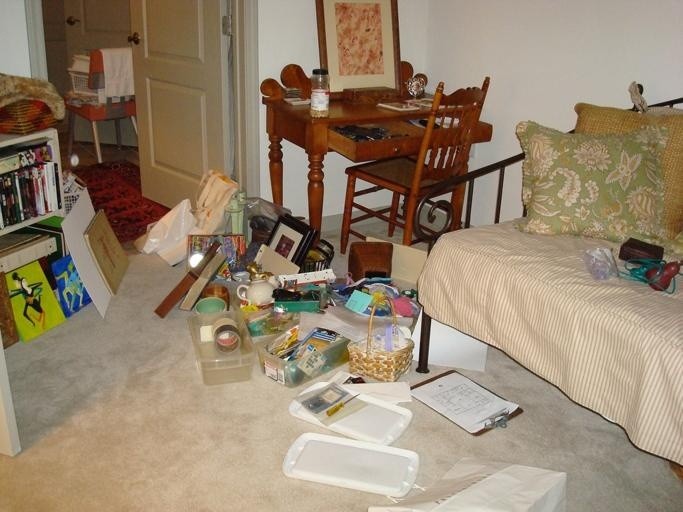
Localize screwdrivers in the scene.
[326,393,361,416]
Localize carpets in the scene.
[73,159,171,243]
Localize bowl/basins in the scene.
[195,296,227,314]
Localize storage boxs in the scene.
[186,311,256,386]
[255,327,350,389]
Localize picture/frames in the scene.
[265,214,319,267]
[315,1,403,101]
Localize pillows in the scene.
[517,121,667,250]
[574,101,683,239]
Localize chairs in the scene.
[63,46,140,166]
[340,76,490,255]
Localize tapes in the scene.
[211,317,239,350]
[213,325,242,354]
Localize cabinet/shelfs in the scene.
[1,128,66,237]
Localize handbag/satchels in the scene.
[193,169,240,234]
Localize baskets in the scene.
[347,296,416,384]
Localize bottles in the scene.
[309,69,331,118]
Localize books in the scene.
[178,253,228,312]
[84,208,130,297]
[0,145,62,231]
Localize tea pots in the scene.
[236,279,280,306]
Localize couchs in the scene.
[413,98,683,469]
